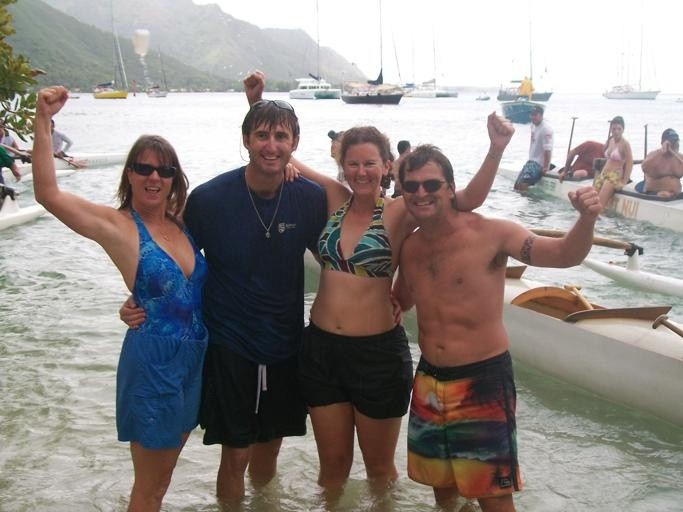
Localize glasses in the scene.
[251,100,294,112]
[131,162,177,178]
[402,179,442,192]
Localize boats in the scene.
[602,85,661,100]
[475,97,489,101]
[401,82,457,98]
[145,89,166,98]
[500,97,546,124]
[0,188,47,232]
[495,86,553,102]
[2,150,130,180]
[67,96,79,100]
[304,240,682,431]
[480,159,682,233]
[340,92,404,105]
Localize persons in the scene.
[641,128,683,198]
[559,136,613,183]
[32,85,209,512]
[514,106,553,190]
[0,119,18,157]
[118,101,401,503]
[0,129,22,211]
[393,144,602,511]
[592,116,632,207]
[243,70,515,490]
[51,119,73,157]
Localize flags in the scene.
[367,71,382,85]
[309,73,319,80]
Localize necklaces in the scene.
[153,218,171,242]
[245,171,285,239]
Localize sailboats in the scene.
[286,1,342,100]
[90,0,129,98]
[335,0,401,95]
